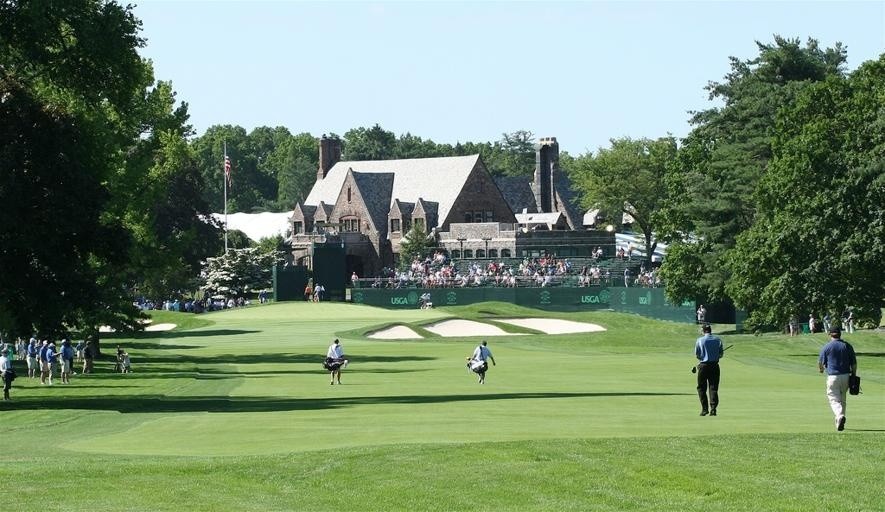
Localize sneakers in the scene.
[709,409,718,417]
[26,370,77,387]
[835,416,846,431]
[699,408,709,416]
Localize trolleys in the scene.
[112,345,133,374]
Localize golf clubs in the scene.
[692,344,733,373]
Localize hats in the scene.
[28,336,69,348]
[701,324,712,331]
[826,325,841,334]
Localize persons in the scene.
[0,337,94,400]
[327,338,345,385]
[258,290,268,304]
[695,324,723,416]
[624,267,631,287]
[617,248,624,260]
[305,283,322,302]
[371,250,571,289]
[696,305,707,324]
[633,264,661,288]
[136,292,252,314]
[351,272,358,286]
[789,309,854,337]
[472,341,495,384]
[818,324,856,431]
[628,241,632,261]
[420,293,431,309]
[578,247,612,288]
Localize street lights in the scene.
[481,237,491,258]
[456,237,467,258]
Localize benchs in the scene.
[456,258,642,287]
